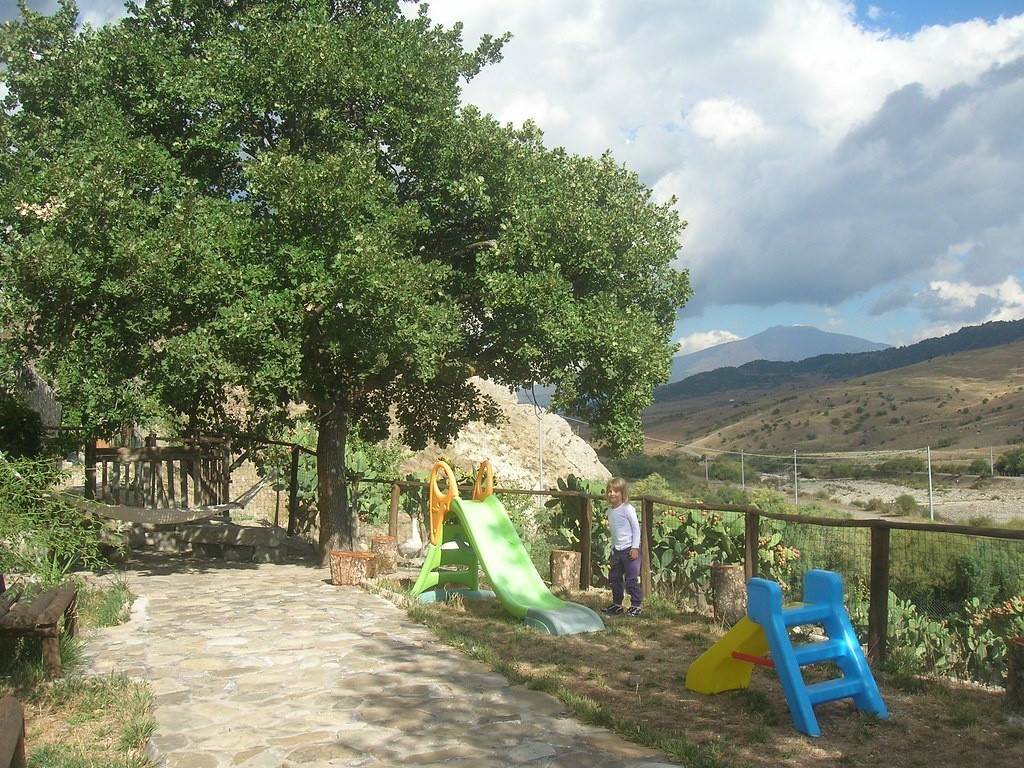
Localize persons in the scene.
[599,478,643,617]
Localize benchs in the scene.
[0,580,78,680]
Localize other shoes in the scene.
[600,603,624,615]
[623,605,642,617]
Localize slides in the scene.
[451,495,607,636]
[686,601,813,695]
